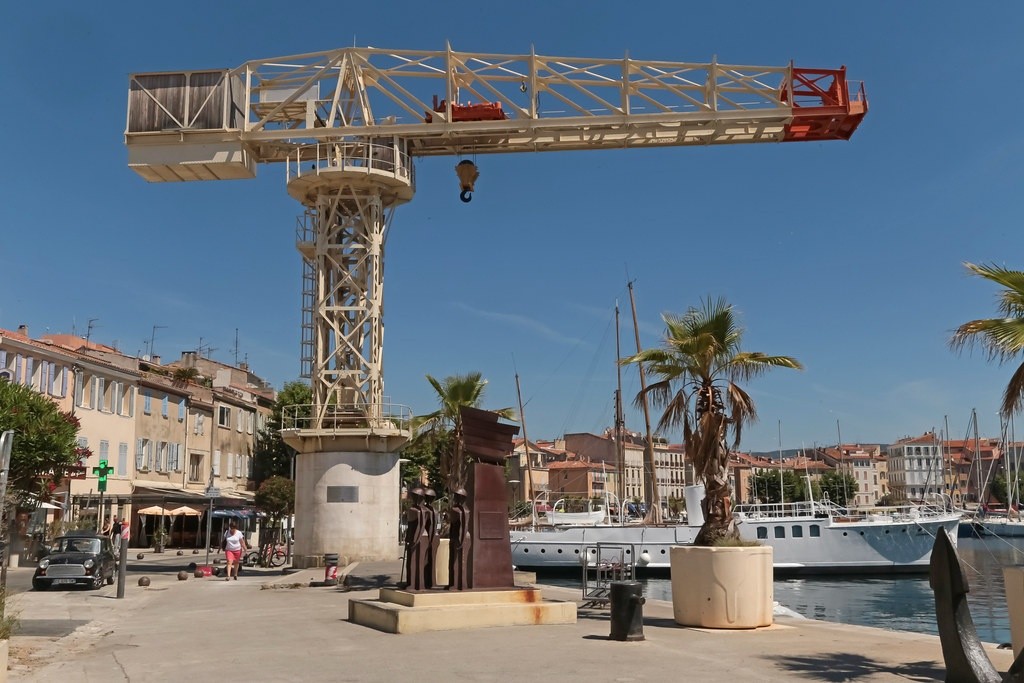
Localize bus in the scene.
[528,499,565,517]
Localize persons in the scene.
[216,522,249,581]
[401,488,472,591]
[100,517,121,553]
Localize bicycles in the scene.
[246,543,287,566]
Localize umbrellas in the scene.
[20,495,62,510]
[136,505,201,546]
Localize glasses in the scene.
[230,527,236,530]
[105,518,108,521]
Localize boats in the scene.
[510,480,964,574]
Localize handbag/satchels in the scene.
[222,531,228,551]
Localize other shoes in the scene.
[226,577,230,581]
[234,576,238,580]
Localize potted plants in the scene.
[154,524,169,553]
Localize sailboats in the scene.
[935,406,1023,536]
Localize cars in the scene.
[32,531,117,589]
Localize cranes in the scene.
[124,37,870,454]
